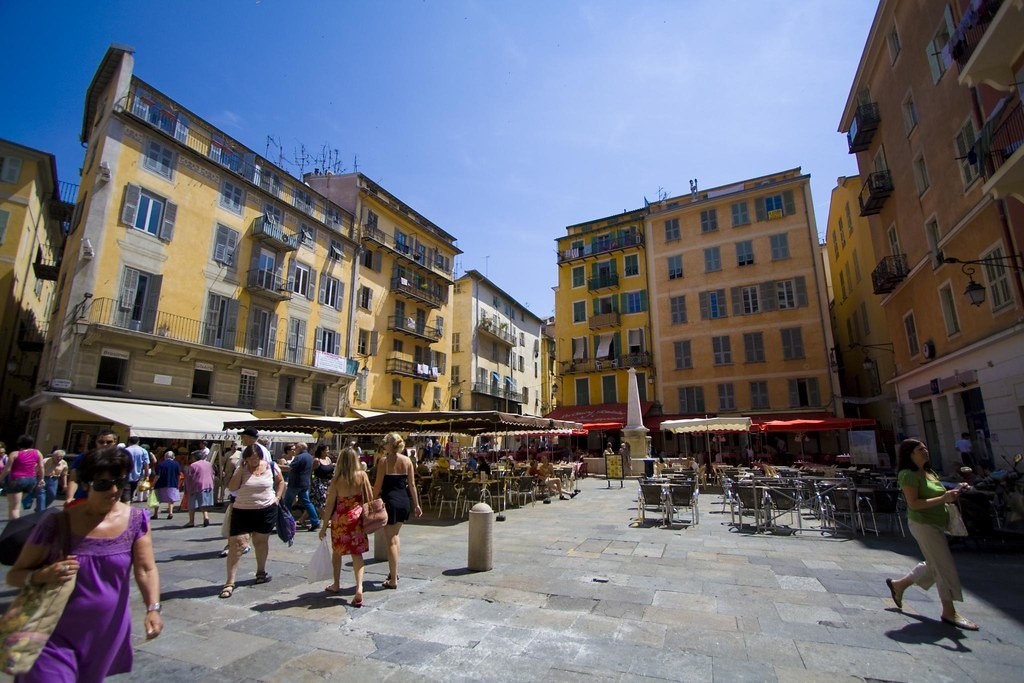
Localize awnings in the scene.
[505,377,515,386]
[659,416,750,434]
[57,395,316,445]
[220,399,653,441]
[750,412,878,433]
[492,373,502,383]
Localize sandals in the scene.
[255,570,267,584]
[219,584,236,598]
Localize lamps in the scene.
[942,252,1024,307]
[848,342,895,372]
[829,348,838,373]
[72,302,93,335]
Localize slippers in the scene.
[326,584,340,593]
[352,594,364,607]
[381,579,398,589]
[388,574,400,580]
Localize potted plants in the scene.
[158,322,170,337]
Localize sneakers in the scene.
[308,524,320,532]
[218,544,231,556]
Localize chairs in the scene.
[637,467,699,528]
[611,359,618,368]
[409,462,587,520]
[596,360,603,370]
[717,465,906,540]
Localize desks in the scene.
[491,468,512,472]
[462,479,500,502]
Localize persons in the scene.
[318,449,374,607]
[656,436,788,464]
[885,438,981,631]
[953,432,977,473]
[6,445,165,683]
[219,444,286,599]
[370,433,422,590]
[0,430,635,534]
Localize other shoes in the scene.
[184,522,194,528]
[300,518,308,529]
[203,518,210,527]
[150,514,158,519]
[167,514,173,519]
[570,492,579,499]
[559,496,569,500]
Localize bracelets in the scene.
[24,570,35,589]
[276,495,282,500]
[145,603,163,613]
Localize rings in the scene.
[60,565,70,573]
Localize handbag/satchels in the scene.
[307,538,333,583]
[146,490,159,508]
[21,495,34,509]
[276,503,298,543]
[0,512,79,675]
[362,497,388,533]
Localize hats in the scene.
[237,427,260,439]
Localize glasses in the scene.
[98,439,114,445]
[87,477,130,491]
